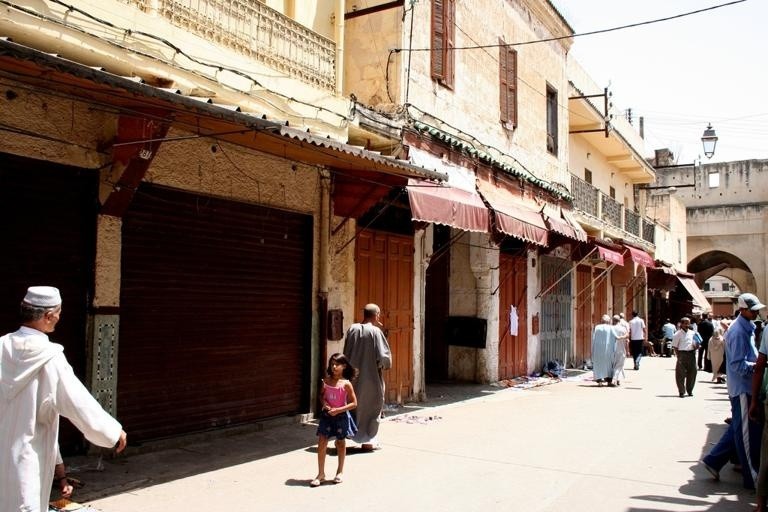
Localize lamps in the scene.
[699,120,721,160]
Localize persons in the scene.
[334,303,392,451]
[309,353,362,487]
[0,285,128,511]
[55,445,74,499]
[591,293,768,491]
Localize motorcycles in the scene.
[662,329,676,357]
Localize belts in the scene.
[679,350,695,353]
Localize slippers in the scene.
[333,475,345,483]
[310,476,327,487]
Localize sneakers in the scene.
[362,442,383,450]
[715,380,723,384]
[607,382,621,387]
[688,394,693,396]
[699,368,704,371]
[698,457,721,480]
[634,363,639,370]
[680,395,684,397]
[598,384,603,387]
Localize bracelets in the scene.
[59,476,67,480]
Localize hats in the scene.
[738,292,767,312]
[22,285,63,310]
